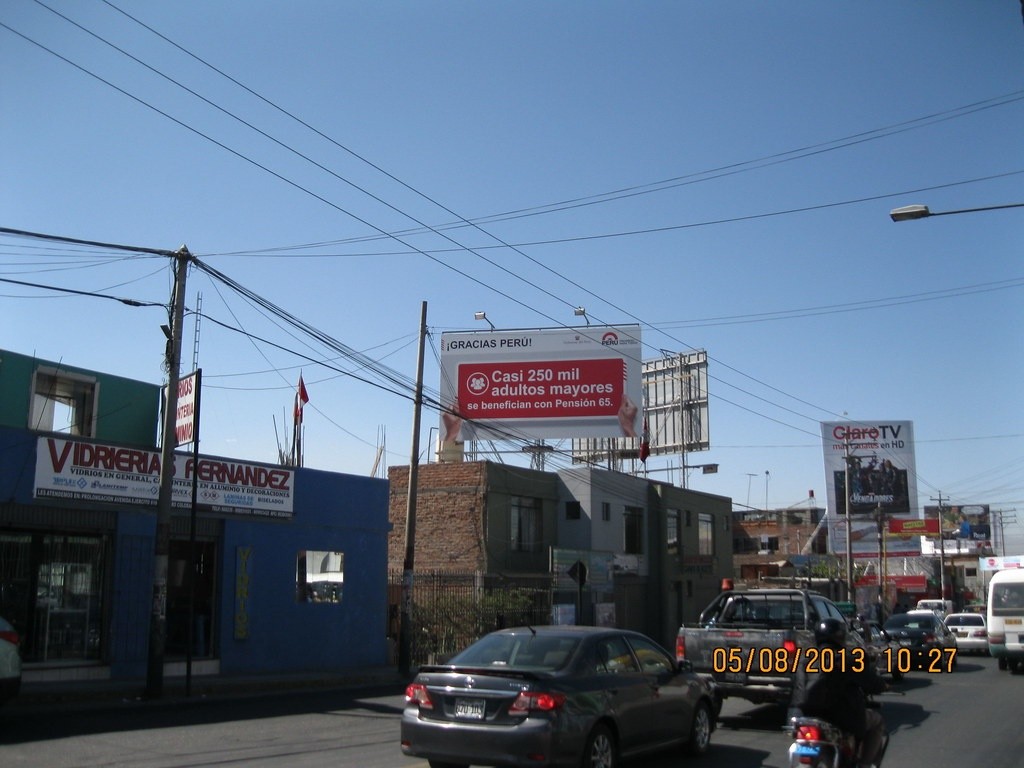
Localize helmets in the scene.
[814,619,847,651]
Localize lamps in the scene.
[574,306,590,325]
[474,311,495,330]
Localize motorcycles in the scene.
[787,678,890,768]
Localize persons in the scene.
[860,617,871,642]
[892,603,909,614]
[802,619,884,768]
[842,459,902,495]
[442,394,638,441]
[959,514,970,537]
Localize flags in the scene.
[297,376,308,414]
[641,419,650,462]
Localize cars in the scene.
[945,613,989,656]
[916,599,952,620]
[881,613,958,671]
[846,618,905,679]
[0,616,22,699]
[400,626,722,768]
[899,610,945,647]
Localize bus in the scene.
[987,569,1024,670]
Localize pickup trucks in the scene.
[674,588,876,714]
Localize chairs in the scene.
[544,651,569,667]
